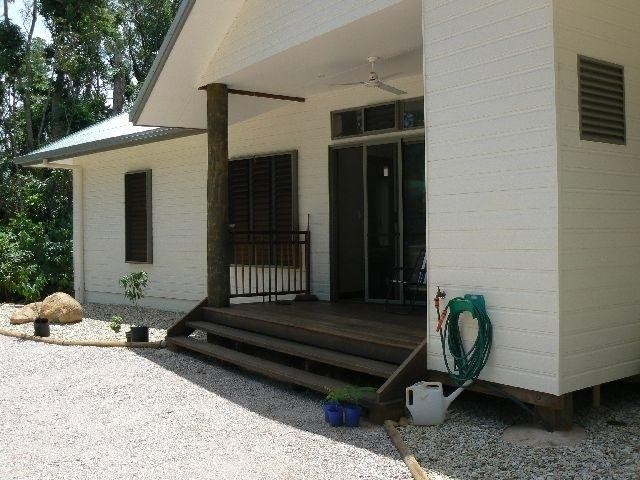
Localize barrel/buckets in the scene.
[324,403,337,421]
[328,408,343,427]
[344,405,362,428]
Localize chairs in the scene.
[384,247,426,312]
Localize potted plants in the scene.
[318,385,377,427]
[108,270,151,342]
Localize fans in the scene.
[327,56,407,96]
